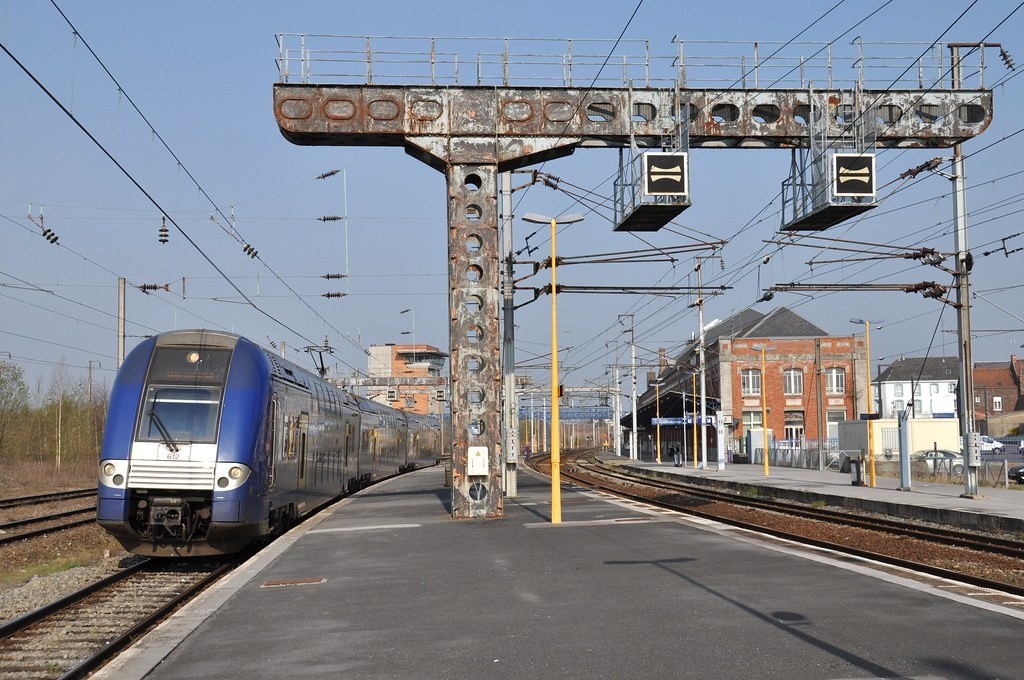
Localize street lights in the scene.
[521,213,585,521]
[749,346,776,475]
[685,371,704,467]
[650,383,665,463]
[850,318,884,486]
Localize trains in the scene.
[95,329,450,563]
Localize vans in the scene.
[959,436,1005,455]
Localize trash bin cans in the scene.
[850,460,865,486]
[674,452,682,467]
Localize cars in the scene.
[908,450,966,478]
[625,442,630,451]
[1008,466,1024,485]
[1017,438,1024,455]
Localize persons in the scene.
[187,413,205,436]
[526,444,531,460]
